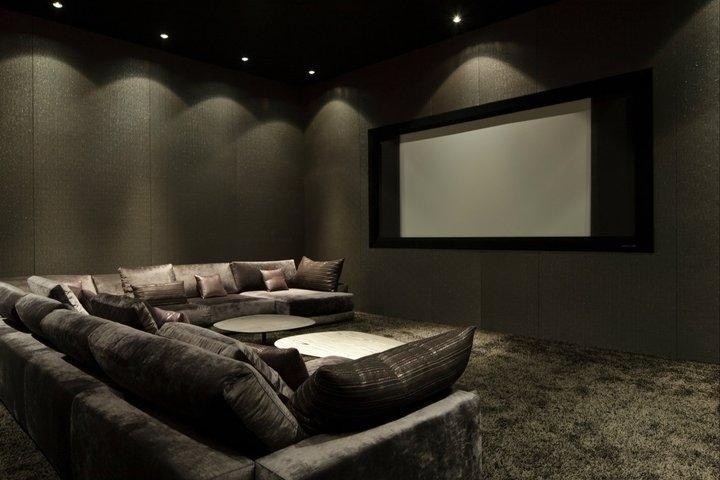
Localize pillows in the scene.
[288,255,345,292]
